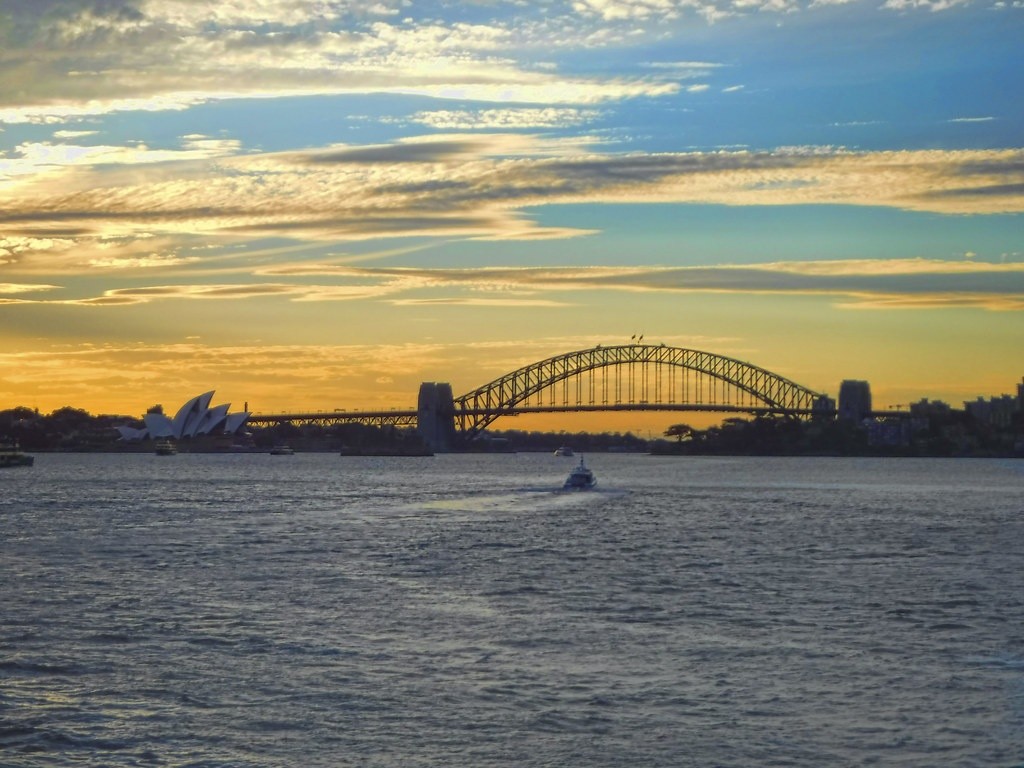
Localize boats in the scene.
[561,454,599,490]
[155,439,178,456]
[270,442,297,455]
[554,445,574,455]
[0,447,38,468]
